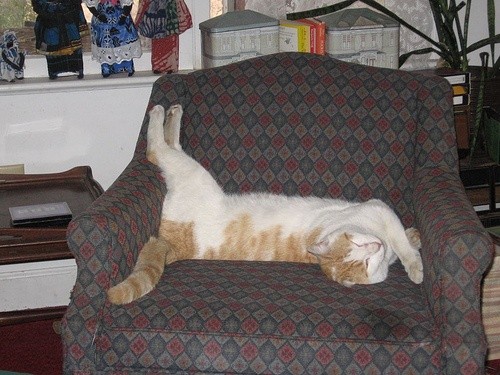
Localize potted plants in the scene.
[286,0,500,157]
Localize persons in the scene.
[0,29,25,83]
[134,0,193,74]
[86,0,143,78]
[31,0,88,80]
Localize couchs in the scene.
[61,51,496,375]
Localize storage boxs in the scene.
[318,9,400,69]
[200,10,281,70]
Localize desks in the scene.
[1,164,103,327]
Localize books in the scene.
[279,18,326,56]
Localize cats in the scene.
[107,104,425,302]
